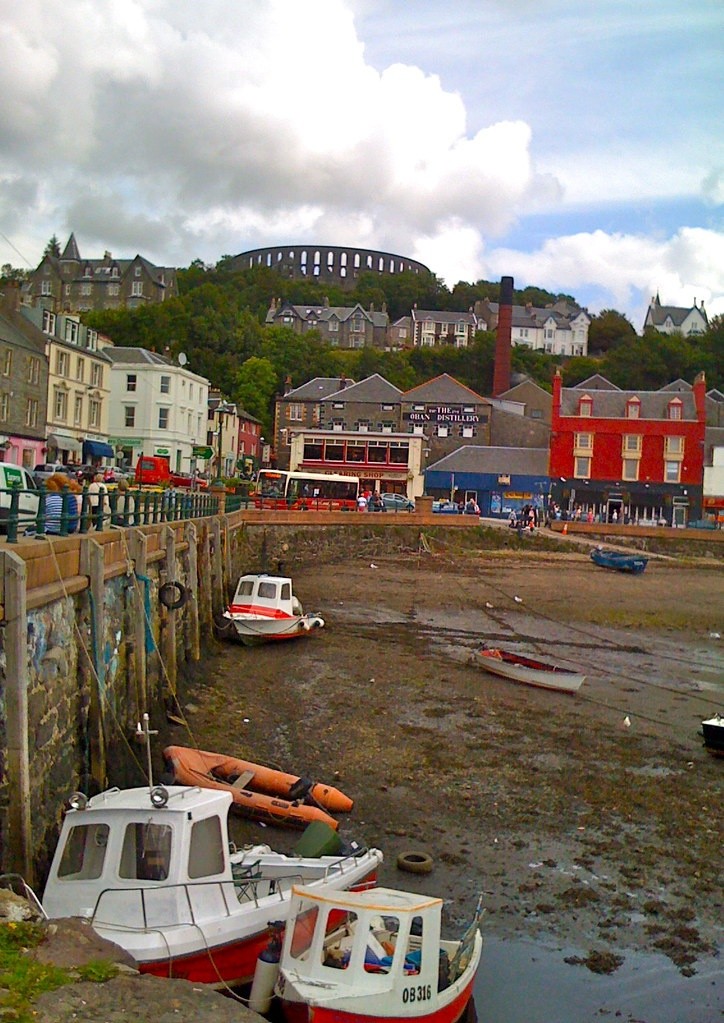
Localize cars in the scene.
[33,463,73,486]
[170,472,207,487]
[72,465,98,487]
[0,462,41,534]
[97,465,130,482]
[688,519,716,529]
[380,492,415,511]
[432,499,460,513]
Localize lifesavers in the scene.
[396,850,434,872]
[160,582,187,610]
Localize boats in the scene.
[247,885,481,1023]
[701,714,724,760]
[590,546,649,573]
[223,574,324,645]
[476,648,587,695]
[40,783,382,989]
[162,746,354,831]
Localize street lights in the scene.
[215,403,229,477]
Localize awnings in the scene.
[48,434,82,452]
[85,440,114,458]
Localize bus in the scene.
[254,468,359,511]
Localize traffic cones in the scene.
[561,521,570,536]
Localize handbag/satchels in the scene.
[476,510,481,514]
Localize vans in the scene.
[136,456,170,486]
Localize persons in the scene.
[509,501,623,531]
[165,482,176,515]
[182,489,192,519]
[457,498,480,516]
[24,468,135,536]
[270,481,354,498]
[357,490,386,513]
